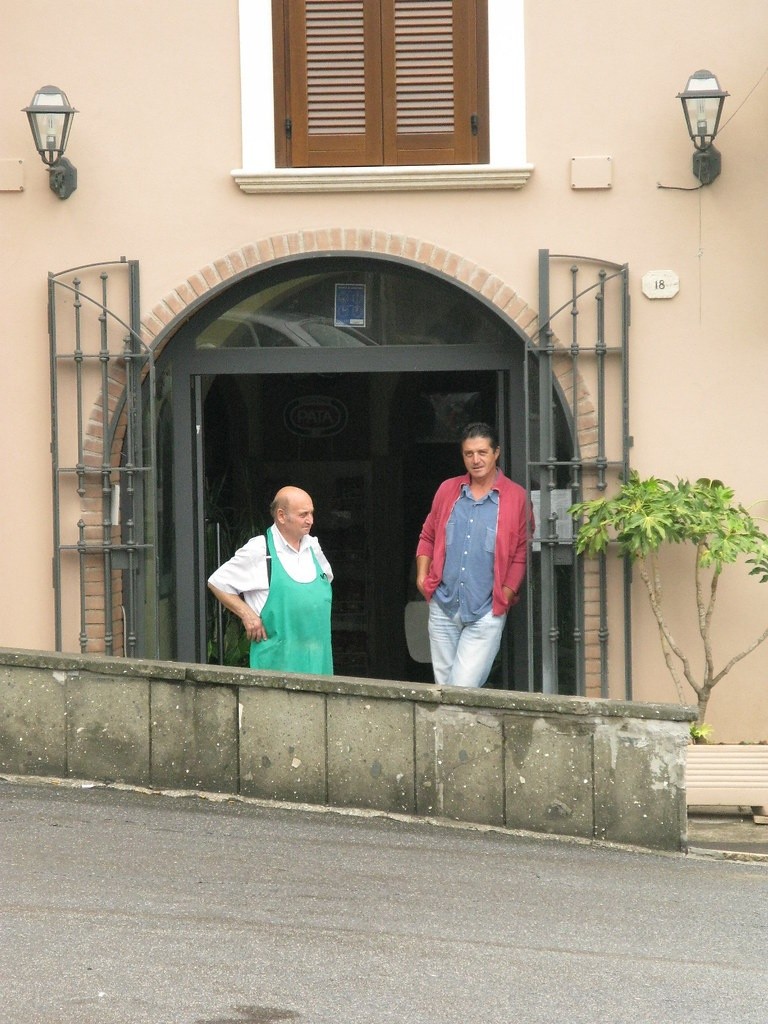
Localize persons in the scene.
[415,422,536,689]
[207,485,334,675]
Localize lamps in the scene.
[20,85,78,201]
[676,68,731,186]
